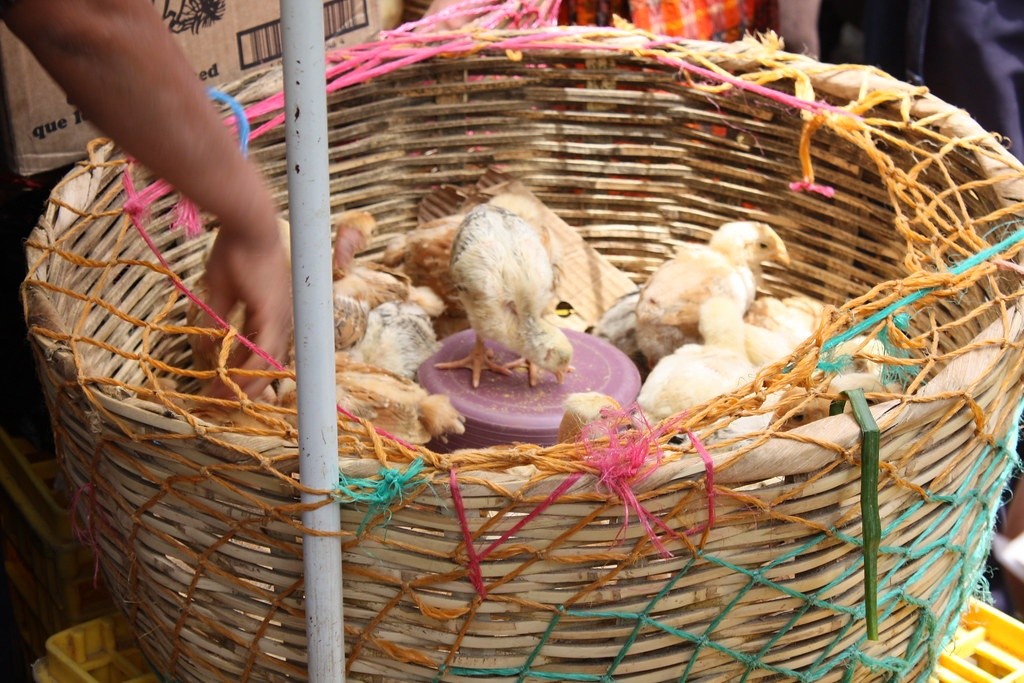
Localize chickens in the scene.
[114,203,891,474]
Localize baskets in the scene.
[18,1,1024,683]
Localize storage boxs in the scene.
[0,0,381,178]
[1,423,1024,682]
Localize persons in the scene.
[1,0,290,433]
